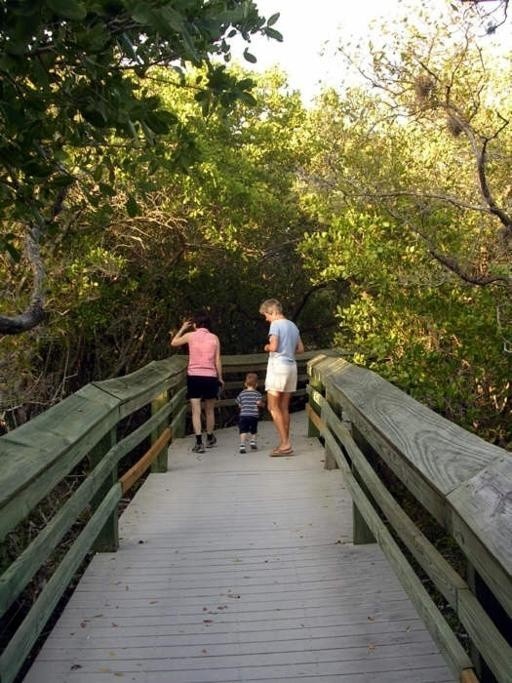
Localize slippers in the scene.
[271,447,293,457]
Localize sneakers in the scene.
[249,440,257,449]
[205,434,216,448]
[192,441,204,453]
[239,445,246,453]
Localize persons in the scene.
[234,373,266,453]
[258,298,305,457]
[171,313,225,453]
